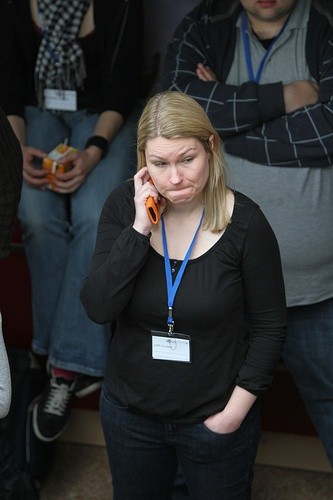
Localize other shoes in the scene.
[32,358,83,443]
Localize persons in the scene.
[78,91,287,500]
[0,0,143,442]
[0,111,31,500]
[150,0,333,474]
